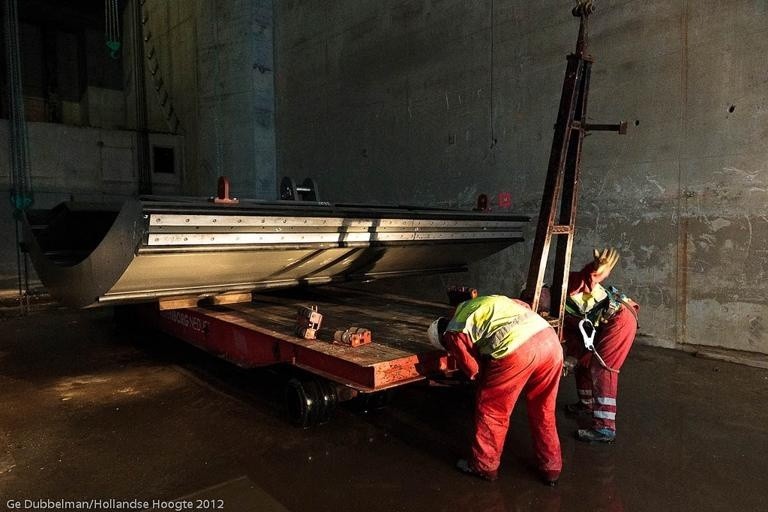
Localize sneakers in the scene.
[456,459,498,482]
[577,428,616,443]
[565,401,592,416]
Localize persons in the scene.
[428,295,565,487]
[519,248,639,442]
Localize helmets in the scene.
[427,317,448,351]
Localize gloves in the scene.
[592,247,620,278]
[563,355,578,377]
